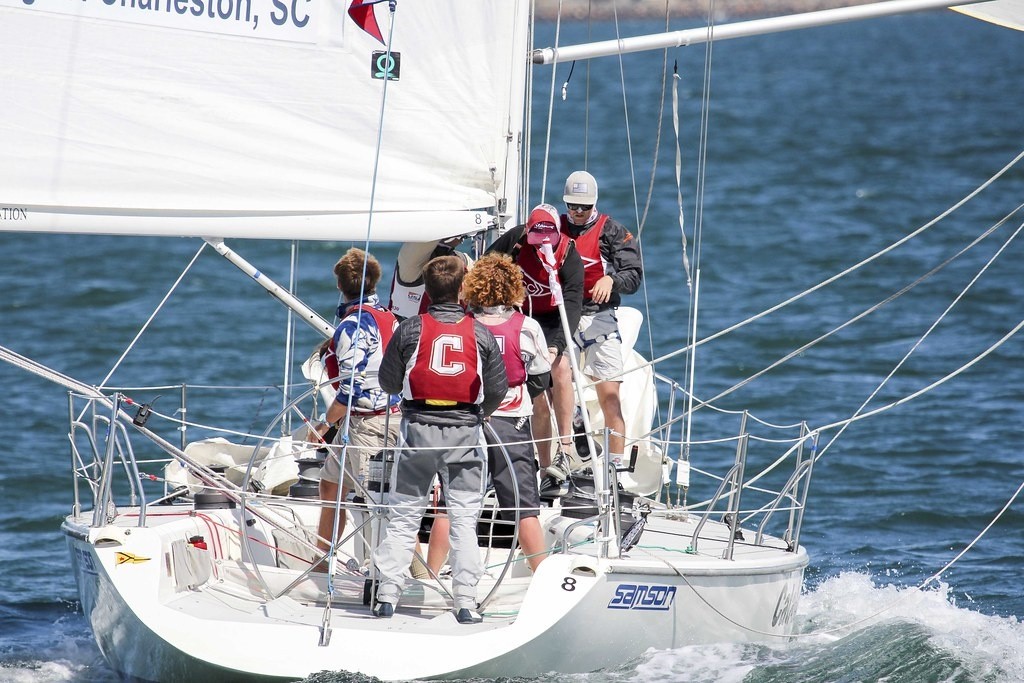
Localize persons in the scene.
[304,171,642,624]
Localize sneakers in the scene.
[545,452,575,481]
[609,464,636,488]
[373,601,396,618]
[573,406,591,460]
[456,608,483,624]
[540,474,561,495]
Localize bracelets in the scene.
[548,348,558,357]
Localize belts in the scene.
[350,405,400,416]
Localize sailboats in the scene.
[1,0,1023,683]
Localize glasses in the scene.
[566,203,594,211]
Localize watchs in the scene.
[319,413,332,429]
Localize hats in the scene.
[563,171,598,205]
[526,203,561,245]
